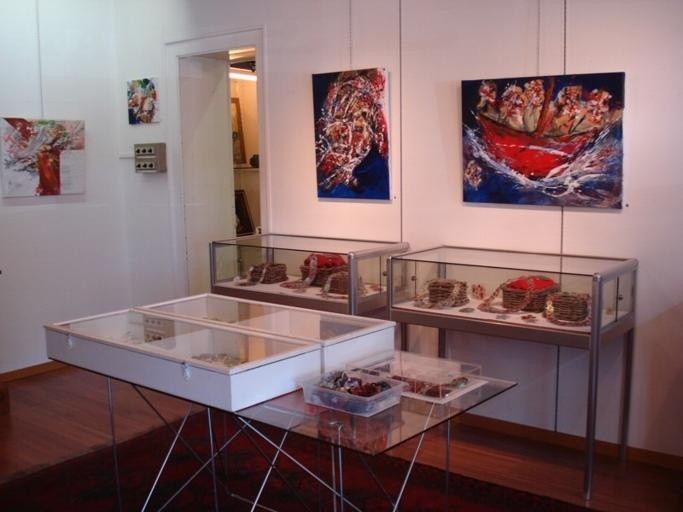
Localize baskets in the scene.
[249,263,288,284]
[325,271,365,296]
[499,275,560,313]
[543,291,592,326]
[425,279,470,308]
[298,263,348,287]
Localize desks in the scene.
[49,350,517,512]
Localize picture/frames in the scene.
[231,97,247,165]
[234,189,256,237]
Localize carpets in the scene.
[0,409,605,512]
